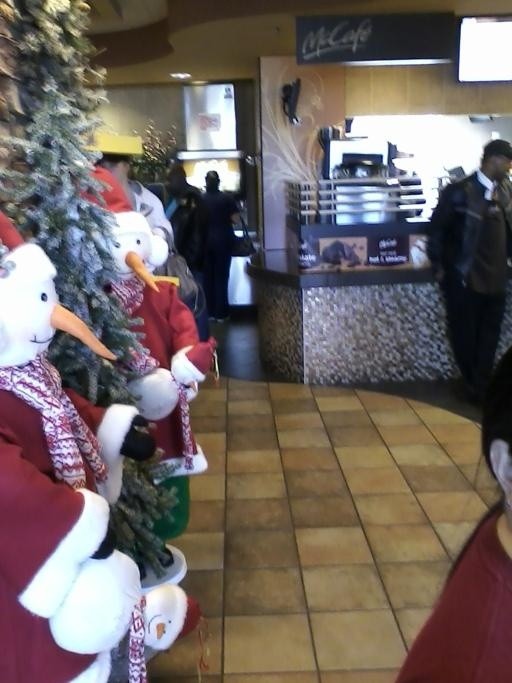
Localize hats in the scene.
[482,139,512,162]
[156,585,206,654]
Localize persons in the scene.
[389,345,511,682]
[424,131,512,406]
[91,153,245,326]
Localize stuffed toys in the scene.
[81,165,218,547]
[0,209,205,682]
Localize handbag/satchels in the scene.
[150,227,208,320]
[229,213,255,258]
